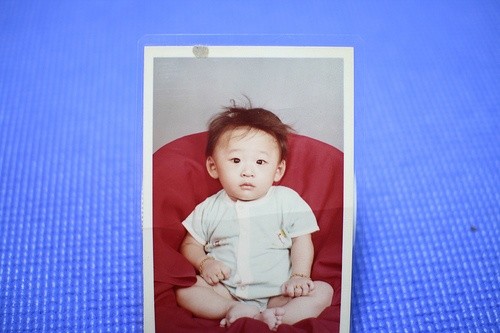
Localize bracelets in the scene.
[289,273,311,280]
[198,254,214,275]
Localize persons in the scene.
[174,96,332,331]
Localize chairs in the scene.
[152,132,344,333]
